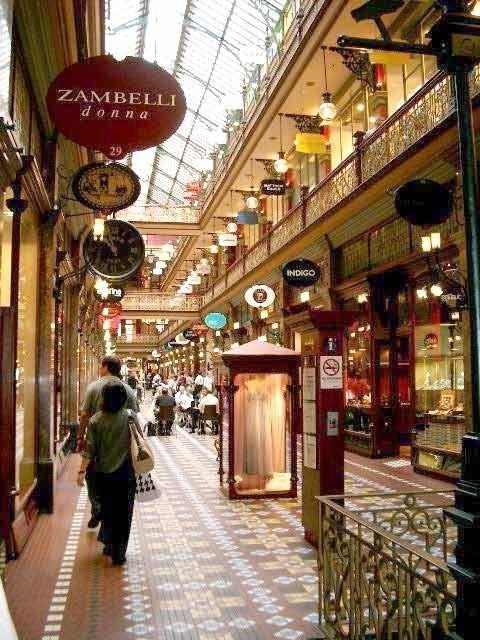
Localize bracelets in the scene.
[78,470,86,475]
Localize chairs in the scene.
[159,405,218,436]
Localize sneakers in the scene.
[88,510,126,565]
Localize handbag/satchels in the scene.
[126,409,154,473]
[134,472,161,503]
[143,421,156,436]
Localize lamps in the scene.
[209,44,379,256]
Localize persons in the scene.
[118,367,223,436]
[76,353,140,543]
[76,380,144,565]
